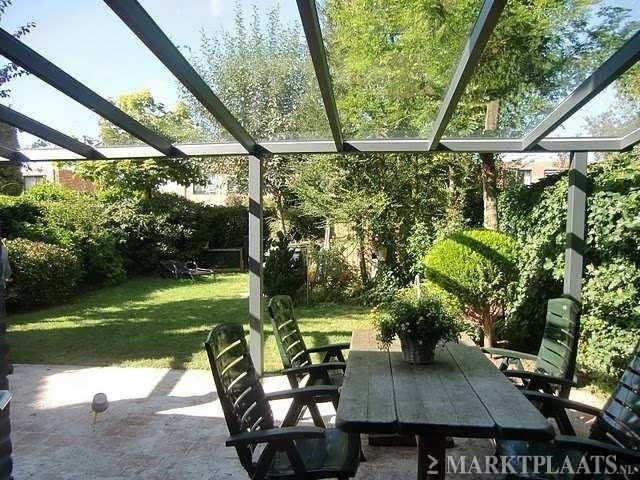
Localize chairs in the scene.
[491,337,640,480]
[173,261,216,281]
[266,294,350,412]
[481,293,583,437]
[205,320,361,480]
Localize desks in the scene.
[337,324,555,480]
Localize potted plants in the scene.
[369,292,472,366]
